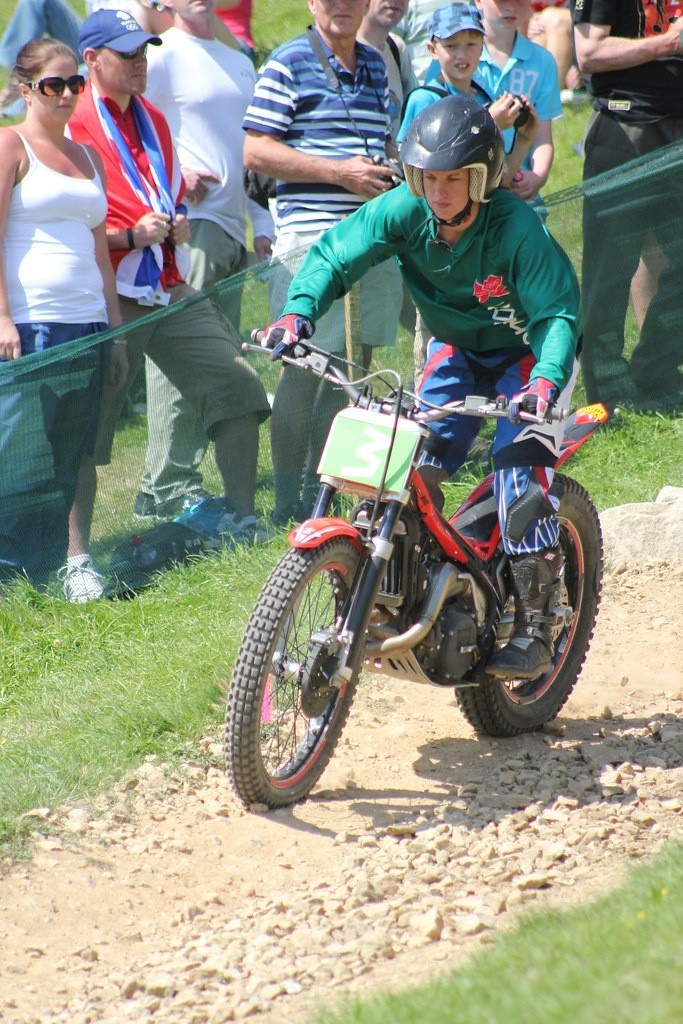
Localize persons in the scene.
[0,0,683,608]
[257,97,582,679]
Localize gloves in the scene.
[507,377,560,425]
[260,312,316,361]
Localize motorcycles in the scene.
[223,330,608,810]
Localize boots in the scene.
[484,547,577,678]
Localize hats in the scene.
[77,8,162,57]
[427,2,487,42]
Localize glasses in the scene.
[28,75,85,96]
[118,44,150,59]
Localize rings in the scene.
[162,221,166,229]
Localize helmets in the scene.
[399,93,507,202]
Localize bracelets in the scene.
[110,339,126,346]
[126,227,134,250]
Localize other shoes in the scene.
[588,397,665,420]
[155,487,218,519]
[209,513,276,551]
[57,559,106,605]
[639,382,683,408]
[131,490,156,519]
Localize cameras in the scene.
[376,158,407,190]
[509,95,531,127]
